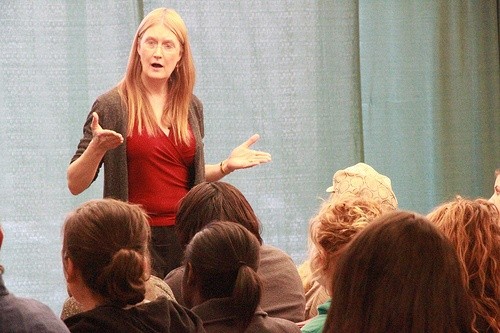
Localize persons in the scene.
[0,163,500,333]
[65,6,272,281]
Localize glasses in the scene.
[145,40,183,53]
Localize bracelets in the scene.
[220,160,234,174]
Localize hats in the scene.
[326,163,398,212]
